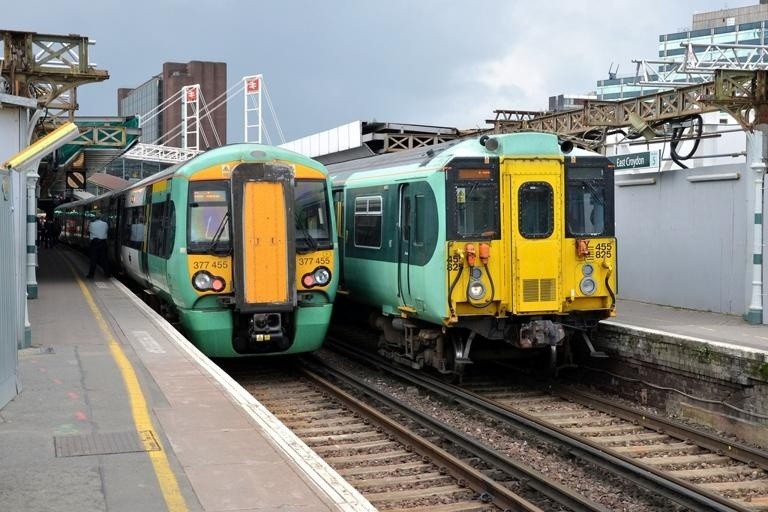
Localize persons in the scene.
[129,216,145,249]
[37,215,62,250]
[85,212,117,280]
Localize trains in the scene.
[324,132,616,384]
[34,144,341,360]
